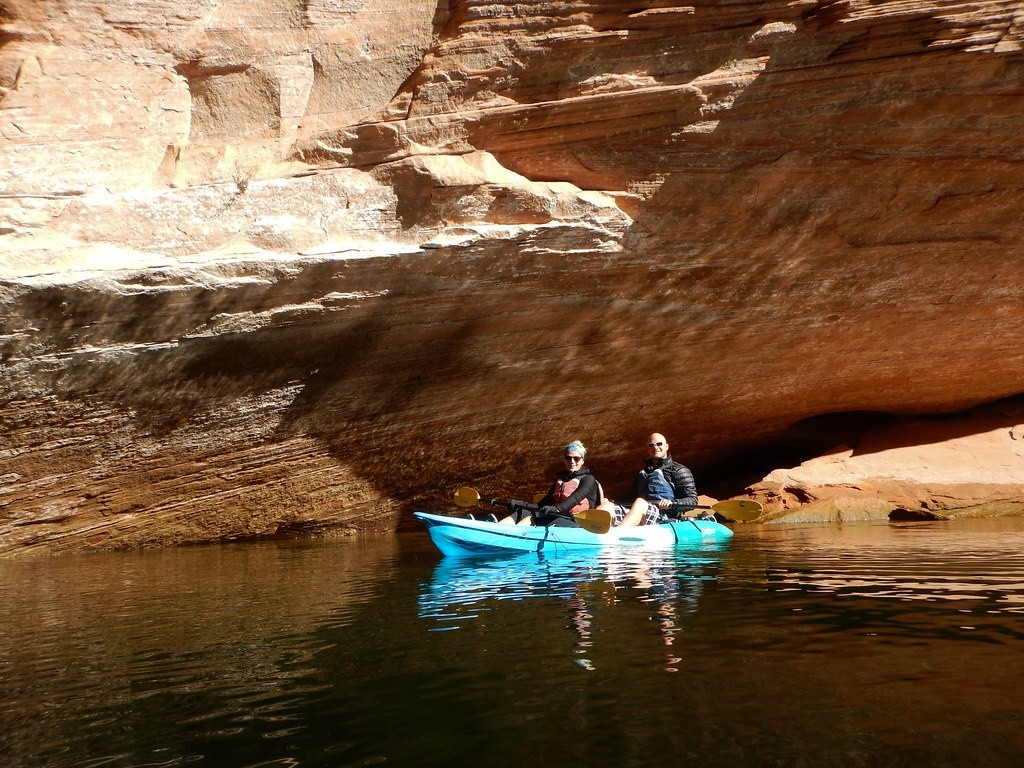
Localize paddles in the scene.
[453,483,611,534]
[654,500,764,521]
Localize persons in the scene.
[465,440,599,527]
[595,432,699,528]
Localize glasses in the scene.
[564,456,583,462]
[648,442,667,448]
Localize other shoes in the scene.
[465,513,475,520]
[486,513,498,523]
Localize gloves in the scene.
[539,505,557,518]
[507,499,527,511]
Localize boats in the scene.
[413,510,731,556]
[418,546,727,617]
